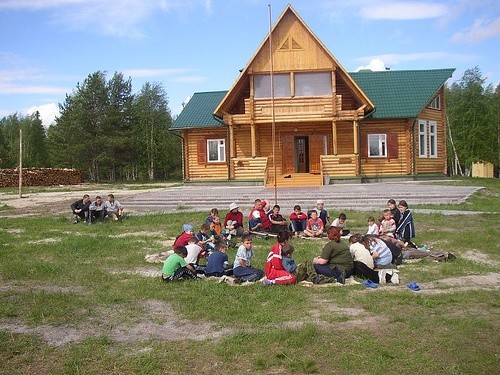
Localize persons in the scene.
[162,198,415,284]
[331,213,350,236]
[204,243,233,277]
[233,235,263,284]
[71,194,123,224]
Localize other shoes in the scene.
[263,279,272,285]
[233,278,243,285]
[378,269,386,284]
[391,273,400,285]
[338,269,346,284]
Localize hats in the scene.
[183,224,193,231]
[229,203,239,212]
[317,200,324,204]
[252,210,261,218]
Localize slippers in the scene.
[363,280,377,288]
[407,282,420,291]
